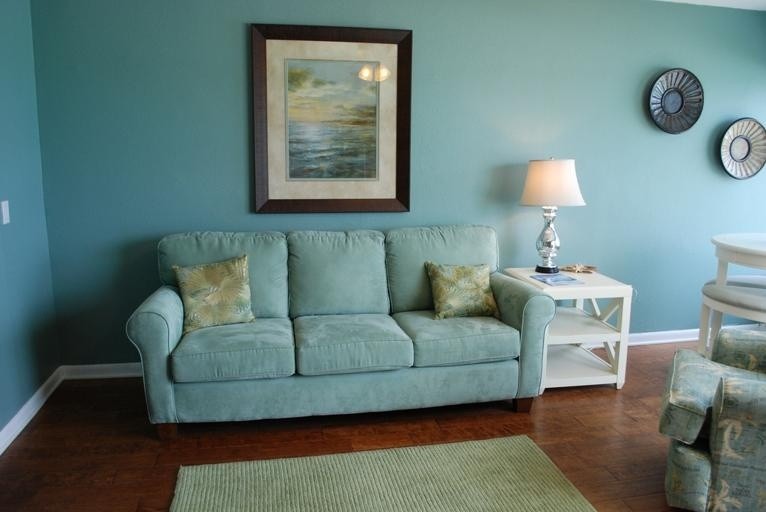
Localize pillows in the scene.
[172,254,257,336]
[426,261,501,318]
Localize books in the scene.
[529,272,586,288]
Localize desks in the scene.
[503,266,632,396]
[699,232,766,355]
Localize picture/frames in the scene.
[250,24,412,213]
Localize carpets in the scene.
[168,434,599,512]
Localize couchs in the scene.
[125,224,555,443]
[659,325,765,512]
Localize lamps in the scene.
[518,158,587,273]
